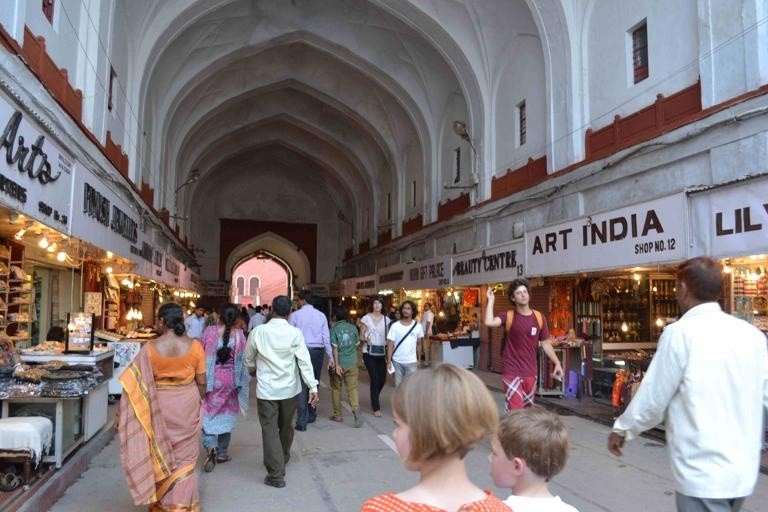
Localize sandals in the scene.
[202,445,219,473]
[217,456,233,464]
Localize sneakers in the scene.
[295,423,307,432]
[329,416,343,422]
[353,408,363,429]
[371,410,382,417]
[264,475,286,488]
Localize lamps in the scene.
[175,175,198,193]
[8,214,67,262]
[338,214,353,228]
[453,120,476,154]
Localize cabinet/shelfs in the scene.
[0,246,38,348]
[0,348,114,468]
[429,336,480,368]
[101,276,119,331]
[573,273,682,342]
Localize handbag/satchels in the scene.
[367,343,386,356]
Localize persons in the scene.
[119,303,206,512]
[362,362,513,512]
[181,288,434,488]
[483,279,563,411]
[488,405,580,512]
[607,257,768,512]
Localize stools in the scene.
[0,417,53,492]
